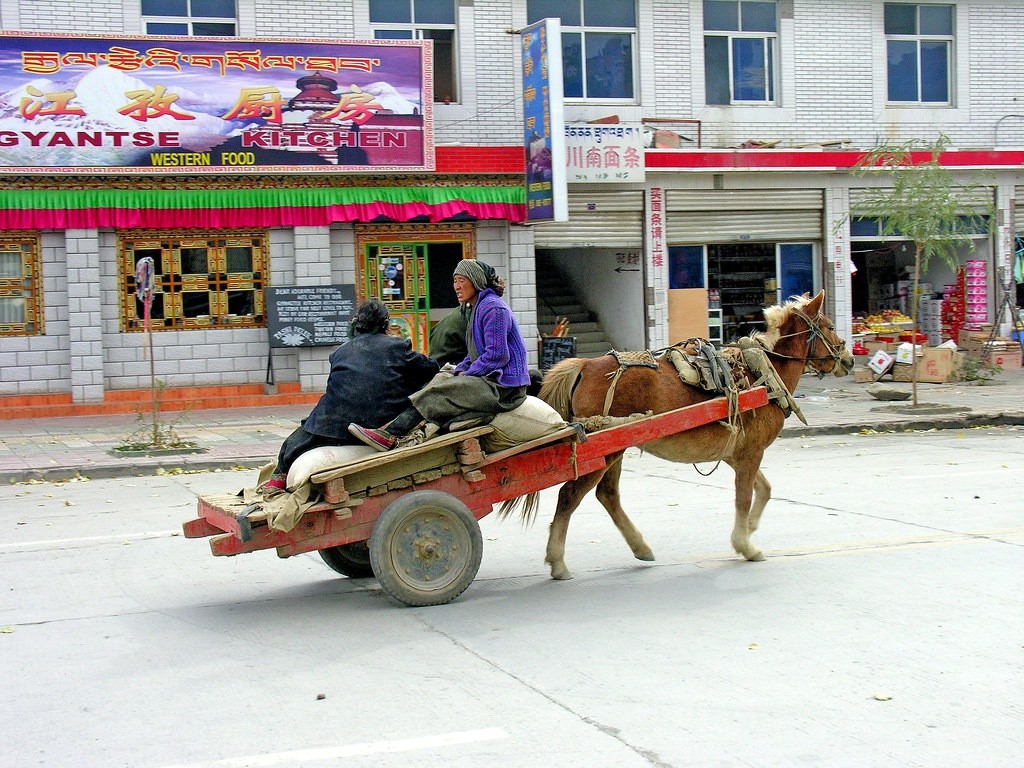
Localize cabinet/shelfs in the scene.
[706,243,777,344]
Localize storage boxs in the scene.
[763,277,777,306]
[849,259,1023,383]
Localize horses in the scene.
[495,289,855,580]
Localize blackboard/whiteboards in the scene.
[542,336,577,367]
[265,283,357,348]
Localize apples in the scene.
[853,310,921,336]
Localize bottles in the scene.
[709,288,720,309]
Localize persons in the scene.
[260,299,442,495]
[348,258,543,452]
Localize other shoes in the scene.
[262,473,288,490]
[348,423,397,452]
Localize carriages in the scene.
[182,292,854,607]
